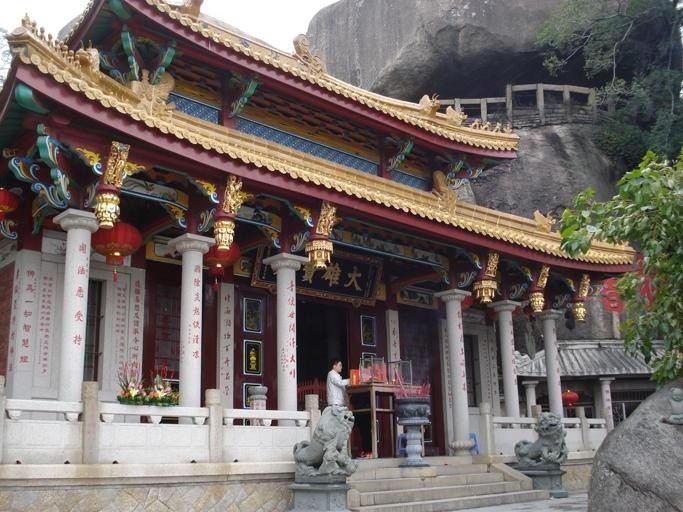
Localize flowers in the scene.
[117,361,182,405]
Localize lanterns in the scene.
[90,221,143,284]
[562,388,579,409]
[461,297,476,318]
[0,190,21,221]
[203,240,241,293]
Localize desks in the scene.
[346,382,425,459]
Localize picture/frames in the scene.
[244,339,263,377]
[361,352,376,369]
[360,315,378,347]
[244,297,263,334]
[243,382,261,409]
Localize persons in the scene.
[325,359,351,406]
